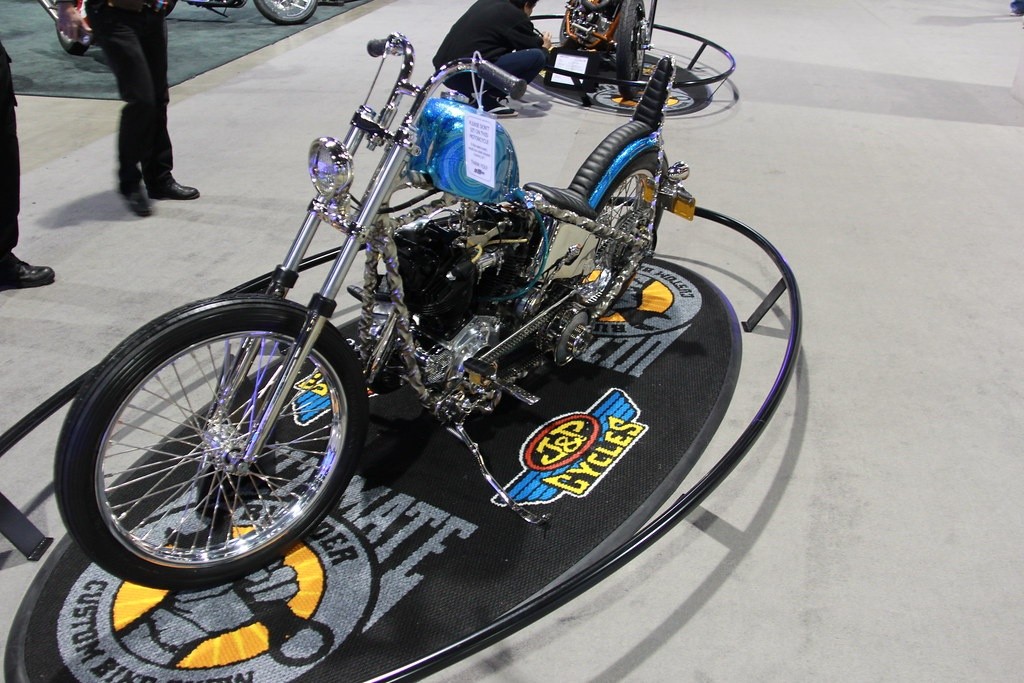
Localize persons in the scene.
[435,1,553,121]
[57,0,201,220]
[0,43,57,291]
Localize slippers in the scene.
[487,106,518,116]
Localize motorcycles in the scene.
[53,33,693,596]
[558,0,658,100]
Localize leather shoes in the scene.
[0,261,55,293]
[148,181,200,200]
[128,192,152,216]
